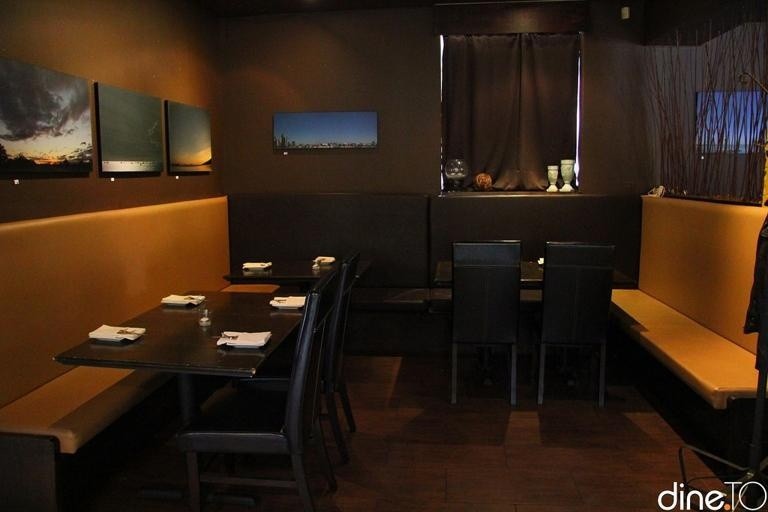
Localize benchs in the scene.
[230,192,430,351]
[426,193,639,336]
[0,192,273,510]
[612,194,768,410]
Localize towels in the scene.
[161,293,206,306]
[313,255,336,263]
[87,322,149,340]
[241,260,272,269]
[216,327,272,349]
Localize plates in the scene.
[160,294,204,307]
[241,261,272,269]
[88,323,145,344]
[218,331,272,349]
[314,256,335,264]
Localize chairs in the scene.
[229,248,361,442]
[452,241,520,406]
[538,243,614,406]
[178,258,344,511]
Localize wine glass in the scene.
[545,165,559,192]
[559,159,576,193]
